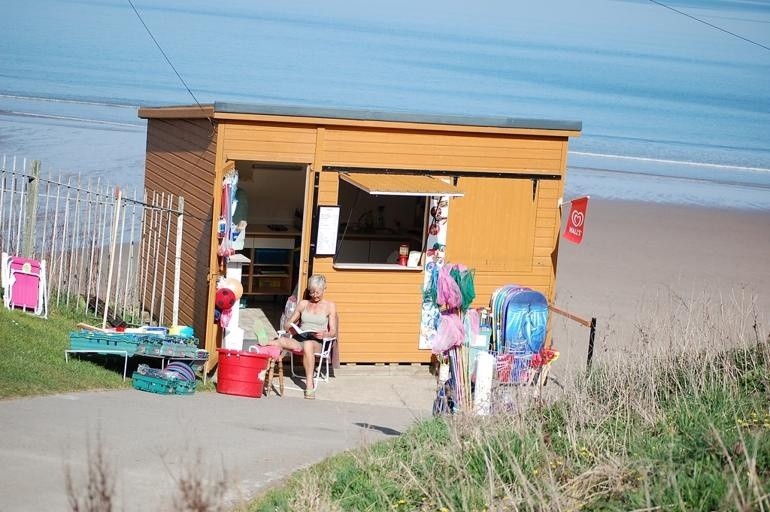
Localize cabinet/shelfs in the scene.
[338,235,409,264]
[235,231,302,295]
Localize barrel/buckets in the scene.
[215,348,272,398]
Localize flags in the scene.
[560,196,589,245]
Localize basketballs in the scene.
[221,278,243,301]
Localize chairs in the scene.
[1,252,48,321]
[272,314,338,391]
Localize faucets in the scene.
[358,212,368,227]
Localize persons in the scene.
[265,271,338,401]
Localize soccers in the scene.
[216,288,235,309]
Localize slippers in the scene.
[305,389,315,399]
[254,319,269,347]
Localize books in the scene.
[289,322,324,346]
